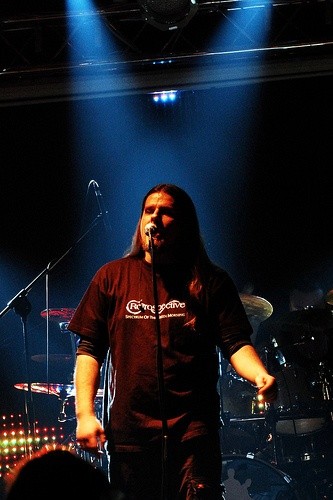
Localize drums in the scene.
[276,418,327,458]
[222,454,301,500]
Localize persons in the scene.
[68,183,279,500]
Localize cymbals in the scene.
[325,290,333,306]
[30,352,77,363]
[12,382,104,398]
[240,290,274,326]
[42,304,79,325]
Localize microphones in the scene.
[144,222,158,237]
[93,181,112,232]
